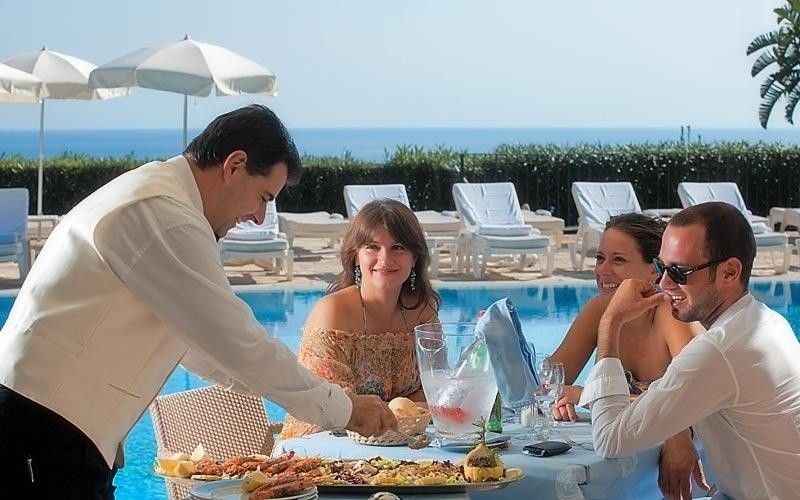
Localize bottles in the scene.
[468,310,503,434]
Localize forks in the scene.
[557,432,593,448]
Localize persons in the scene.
[0,103,400,500]
[533,211,707,424]
[278,198,449,442]
[578,200,800,500]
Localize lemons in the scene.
[241,470,267,493]
[157,443,209,477]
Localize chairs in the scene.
[342,183,439,279]
[218,199,293,282]
[571,181,643,274]
[149,384,285,499]
[0,187,31,287]
[677,182,791,275]
[452,183,555,281]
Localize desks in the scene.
[278,212,349,284]
[412,210,471,275]
[522,209,565,250]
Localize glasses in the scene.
[652,256,744,286]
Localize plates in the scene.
[187,479,318,500]
[442,442,505,454]
[158,474,526,494]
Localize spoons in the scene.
[390,427,430,450]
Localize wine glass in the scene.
[516,352,566,443]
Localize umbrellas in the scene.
[3,60,52,109]
[0,44,132,218]
[86,32,281,154]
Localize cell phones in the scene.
[521,441,571,457]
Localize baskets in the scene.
[345,406,432,446]
